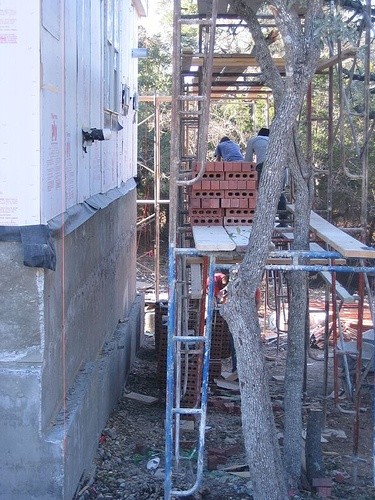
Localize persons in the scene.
[213,136,244,162]
[243,128,289,228]
[205,268,262,380]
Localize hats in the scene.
[258,128,269,136]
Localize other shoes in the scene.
[276,224,288,228]
